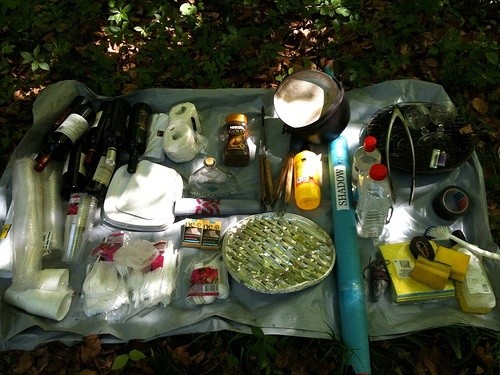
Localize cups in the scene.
[0,155,98,321]
[293,149,323,210]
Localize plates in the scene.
[100,160,184,231]
[360,99,477,174]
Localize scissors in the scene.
[272,129,296,206]
[258,104,274,204]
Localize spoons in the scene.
[82,230,231,325]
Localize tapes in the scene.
[163,102,201,163]
[409,236,434,261]
[433,185,471,221]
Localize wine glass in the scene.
[403,100,437,146]
[429,99,457,147]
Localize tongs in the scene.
[385,107,416,206]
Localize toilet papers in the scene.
[169,102,201,134]
[162,123,195,163]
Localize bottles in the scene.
[33,93,153,214]
[354,163,393,239]
[351,135,382,196]
[187,156,242,199]
[449,229,496,314]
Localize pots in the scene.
[274,70,350,146]
